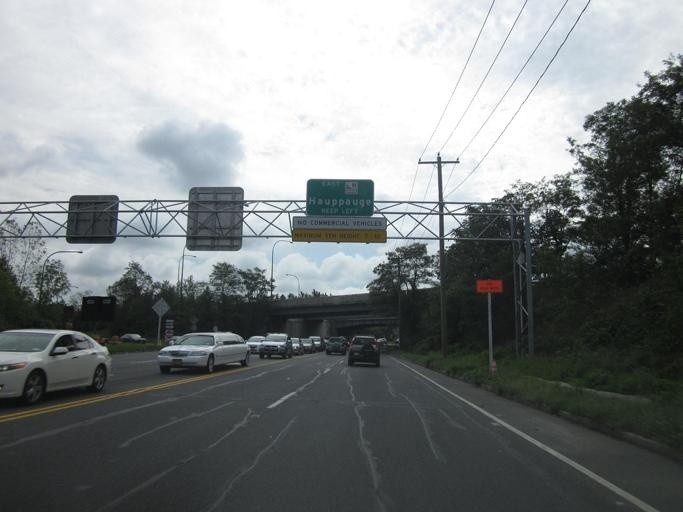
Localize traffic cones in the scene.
[488,360,497,381]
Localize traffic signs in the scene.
[306,180,374,216]
[294,215,387,229]
[291,228,388,244]
[476,280,503,293]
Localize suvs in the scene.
[347,336,380,366]
[260,334,294,358]
[326,336,350,355]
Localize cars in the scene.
[246,336,265,354]
[158,332,251,373]
[291,336,327,356]
[120,333,147,344]
[0,329,112,404]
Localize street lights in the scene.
[286,274,300,299]
[178,253,196,299]
[271,240,293,299]
[39,250,84,317]
